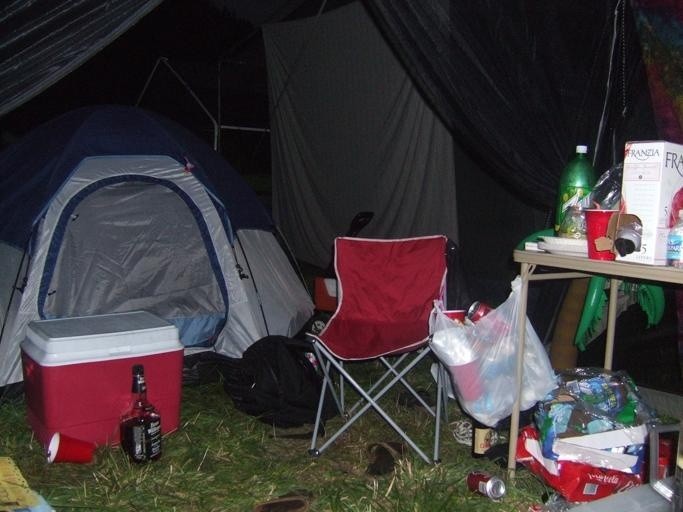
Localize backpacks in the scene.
[216,332,341,431]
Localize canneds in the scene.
[467,301,509,335]
[466,470,506,499]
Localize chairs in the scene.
[303,232,449,467]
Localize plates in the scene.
[536,236,588,258]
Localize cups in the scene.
[444,353,483,399]
[439,310,466,326]
[48,431,96,464]
[584,208,619,261]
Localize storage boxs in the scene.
[19,308,185,453]
[615,140,683,265]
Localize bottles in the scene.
[119,363,163,465]
[555,144,596,238]
[471,421,499,459]
[667,208,683,270]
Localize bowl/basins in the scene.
[558,205,587,237]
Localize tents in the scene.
[259,1,683,417]
[0,104,317,402]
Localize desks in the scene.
[505,249,682,487]
